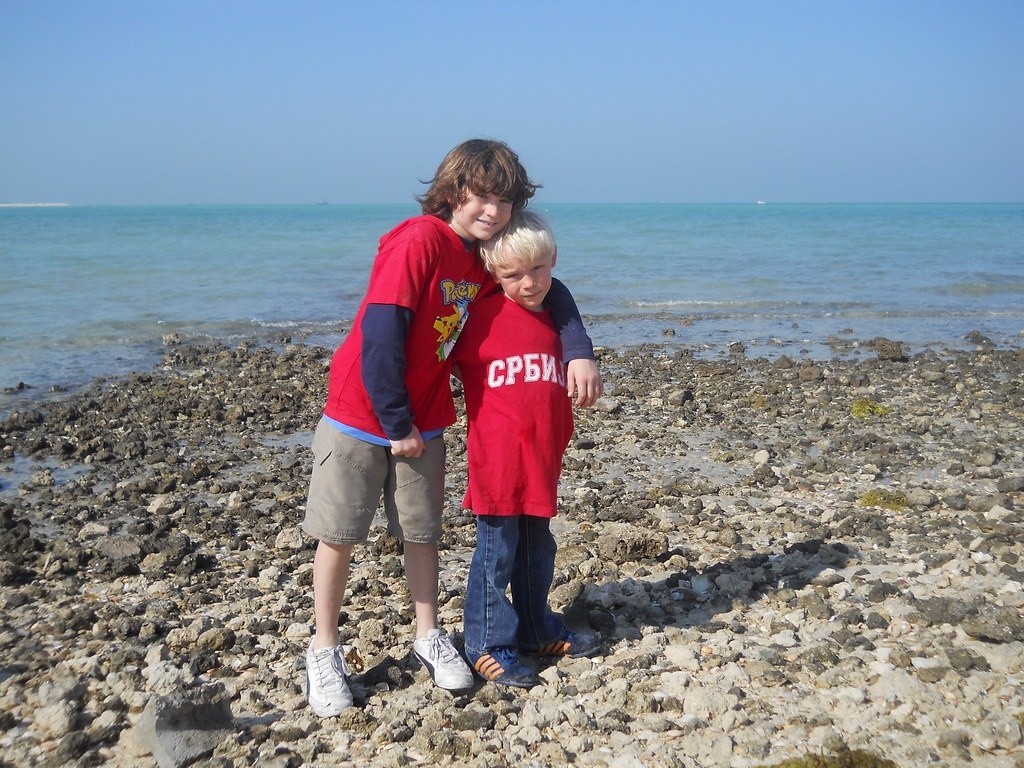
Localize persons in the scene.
[448,211,600,687]
[303,141,602,718]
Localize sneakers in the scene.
[305,635,352,718]
[465,647,540,687]
[412,628,473,689]
[526,630,603,658]
